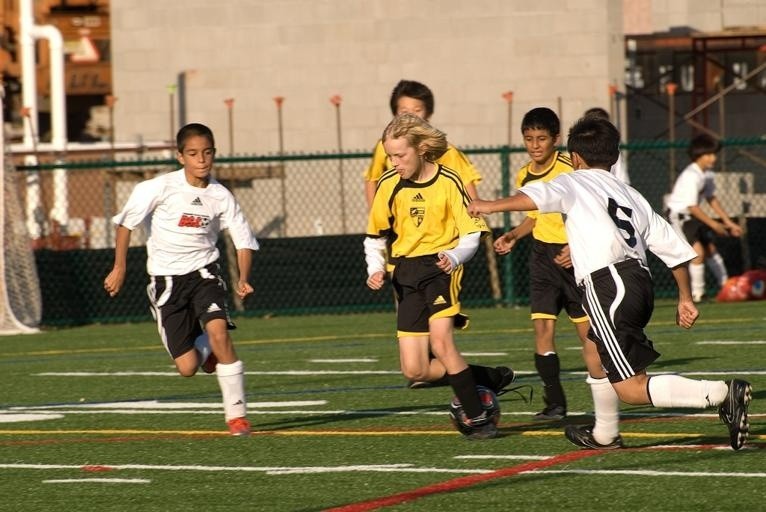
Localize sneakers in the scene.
[535,403,566,420]
[692,292,709,303]
[565,423,623,449]
[715,378,753,451]
[494,366,515,395]
[203,353,216,373]
[228,417,249,436]
[481,422,498,438]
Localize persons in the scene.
[496,106,591,423]
[666,134,745,304]
[105,122,259,438]
[363,115,513,441]
[469,115,752,452]
[366,79,488,329]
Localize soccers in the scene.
[448,385,501,437]
